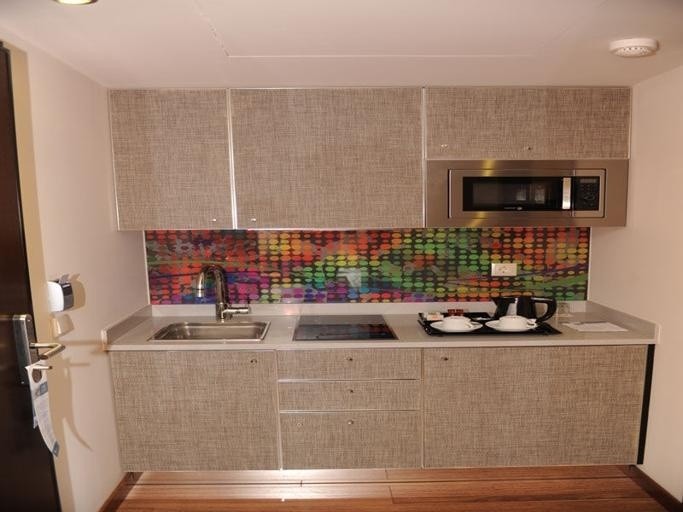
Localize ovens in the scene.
[426,159,629,227]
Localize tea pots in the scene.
[491,295,556,329]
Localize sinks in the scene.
[146,319,273,342]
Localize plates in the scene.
[430,321,483,332]
[486,320,530,332]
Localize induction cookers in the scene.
[292,315,399,342]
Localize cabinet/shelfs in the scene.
[107,88,423,234]
[109,345,647,474]
[422,85,630,159]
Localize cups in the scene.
[499,315,536,329]
[443,315,474,329]
[556,302,569,316]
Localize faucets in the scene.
[194,264,250,323]
